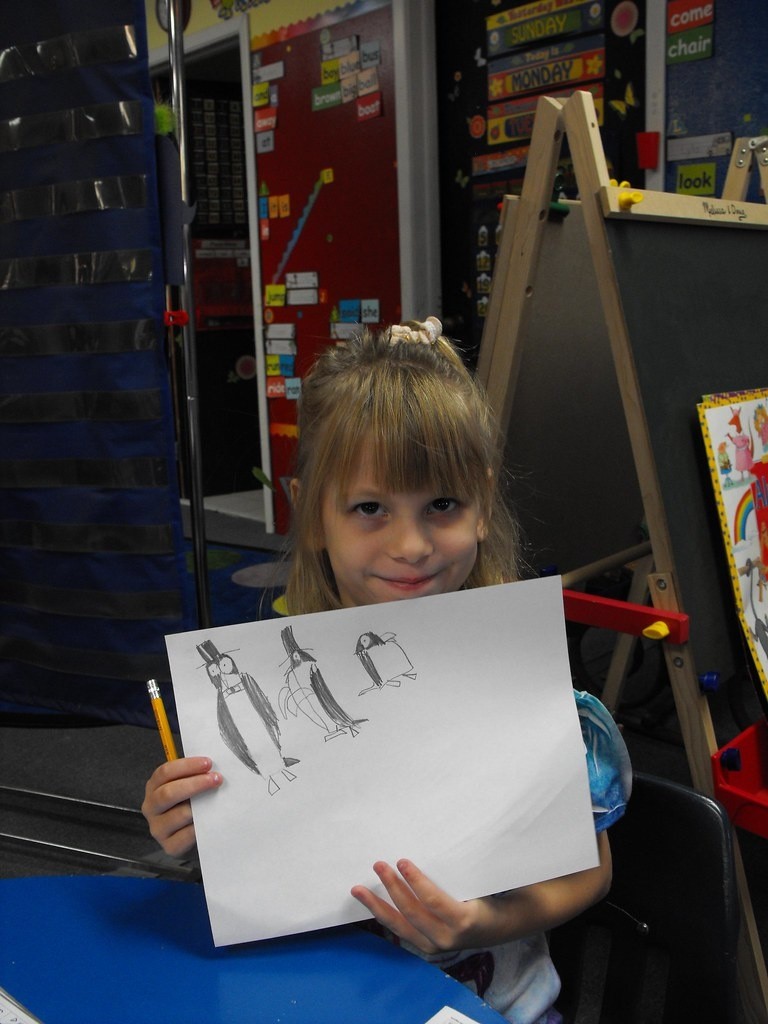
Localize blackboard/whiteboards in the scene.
[583,184,768,700]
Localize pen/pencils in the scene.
[145,678,179,762]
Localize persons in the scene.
[141,316,632,1024]
